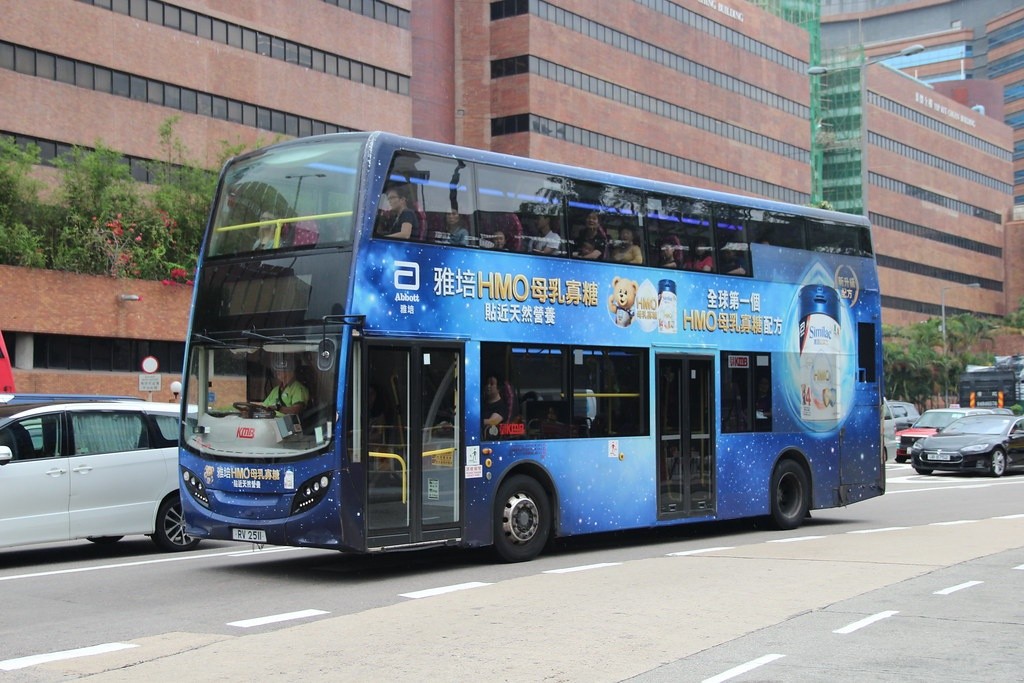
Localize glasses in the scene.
[259,217,273,221]
[386,196,399,200]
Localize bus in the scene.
[176,129,887,568]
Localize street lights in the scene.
[283,174,325,225]
[940,283,981,407]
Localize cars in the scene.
[882,400,922,464]
[0,391,202,562]
[895,408,996,465]
[911,414,1024,477]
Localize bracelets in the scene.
[276,404,280,411]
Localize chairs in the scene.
[483,376,514,441]
[295,364,319,434]
[280,201,683,271]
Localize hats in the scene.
[273,358,296,370]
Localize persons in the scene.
[256,184,772,278]
[248,353,773,512]
